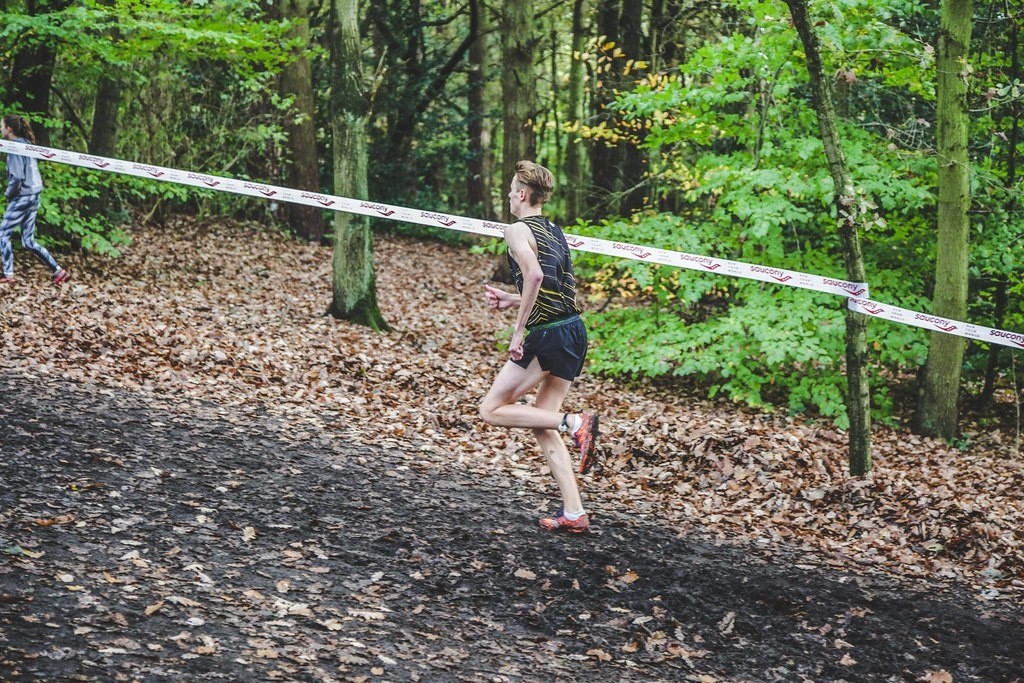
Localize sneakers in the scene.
[571,412,598,475]
[538,509,590,534]
[0,277,17,284]
[51,268,71,285]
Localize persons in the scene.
[480,161,598,536]
[0,114,70,284]
